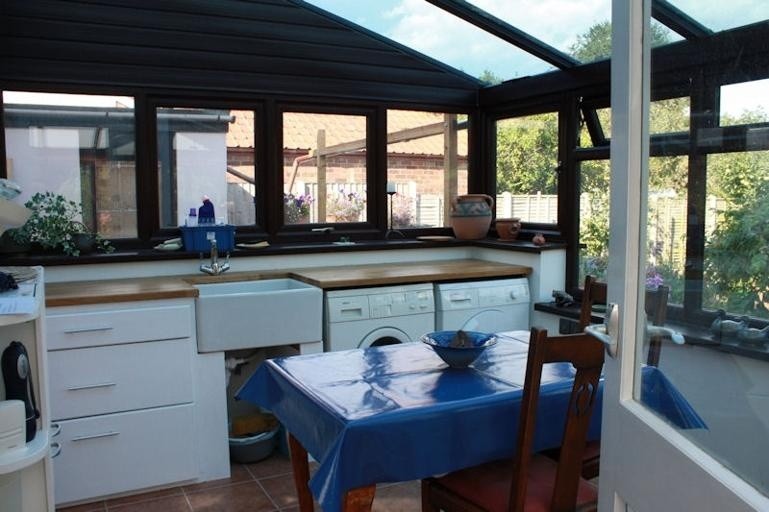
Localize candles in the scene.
[387,181,395,192]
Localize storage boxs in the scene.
[179,225,237,253]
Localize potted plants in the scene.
[5,190,116,257]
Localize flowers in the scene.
[283,190,315,223]
[325,188,366,221]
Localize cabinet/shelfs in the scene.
[1,312,54,512]
[43,296,232,509]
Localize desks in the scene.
[234,330,708,511]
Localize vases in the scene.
[325,215,360,224]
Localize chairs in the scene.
[542,274,669,478]
[422,325,607,511]
